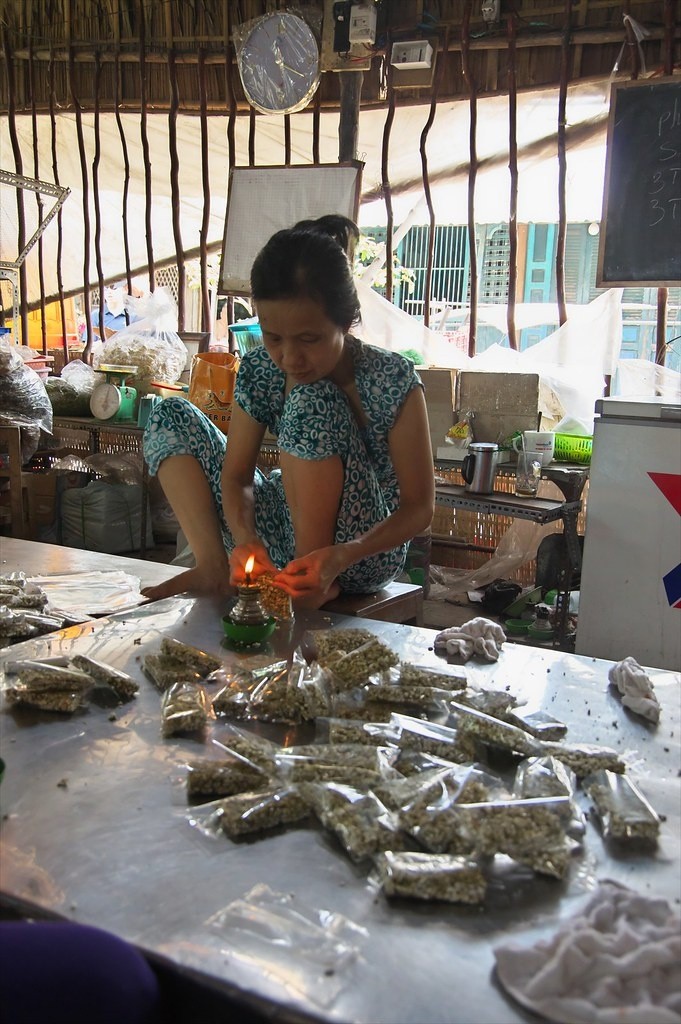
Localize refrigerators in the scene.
[577,395,681,672]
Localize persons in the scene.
[139,213,436,609]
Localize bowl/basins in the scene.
[501,586,542,618]
[544,589,559,604]
[222,614,277,643]
[505,619,557,639]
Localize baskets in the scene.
[22,354,54,380]
[552,432,593,466]
[228,324,264,357]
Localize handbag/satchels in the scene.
[188,353,238,435]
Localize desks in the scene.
[0,536,681,1024]
[29,413,282,543]
[432,456,591,654]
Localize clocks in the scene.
[240,14,321,114]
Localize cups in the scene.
[461,442,499,495]
[514,451,544,499]
[512,430,555,468]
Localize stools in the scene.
[319,580,425,628]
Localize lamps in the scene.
[222,554,278,650]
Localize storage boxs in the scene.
[414,370,559,463]
[25,350,95,384]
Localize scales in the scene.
[88,363,139,424]
